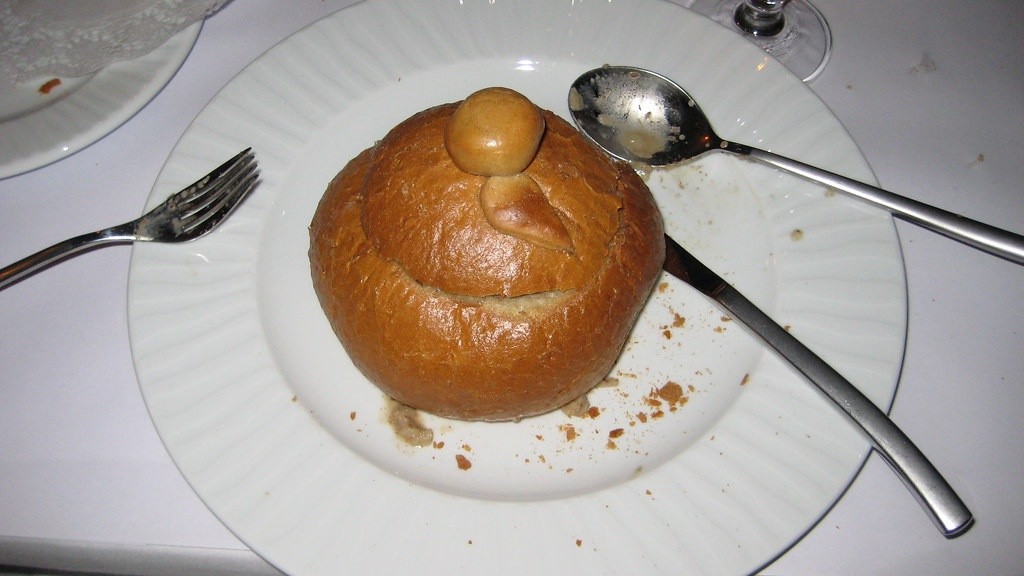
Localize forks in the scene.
[0,148,260,291]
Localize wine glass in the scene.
[685,0,833,84]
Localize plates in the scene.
[0,20,203,180]
[127,0,906,576]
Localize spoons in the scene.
[567,67,1024,267]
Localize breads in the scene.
[307,87,666,422]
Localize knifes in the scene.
[662,234,975,538]
